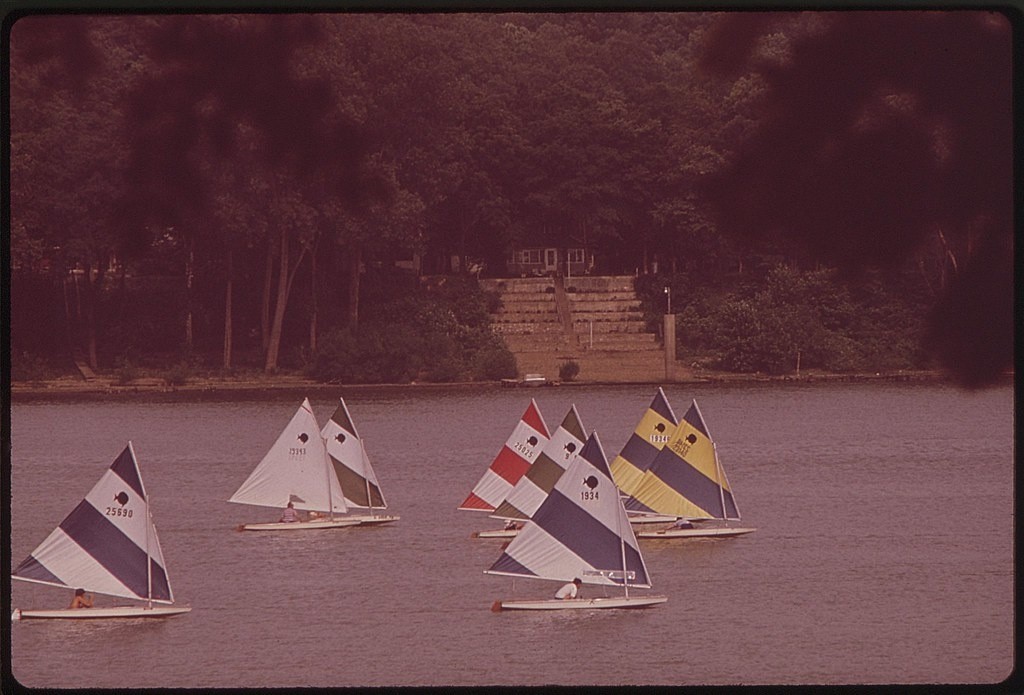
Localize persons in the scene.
[555,578,582,600]
[69,588,95,609]
[667,517,693,530]
[279,502,301,523]
[504,520,517,530]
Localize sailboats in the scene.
[481,427,670,611]
[308,397,401,523]
[455,397,551,539]
[478,403,592,542]
[607,387,707,523]
[618,397,759,539]
[10,441,192,619]
[226,396,362,531]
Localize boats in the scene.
[518,377,545,388]
